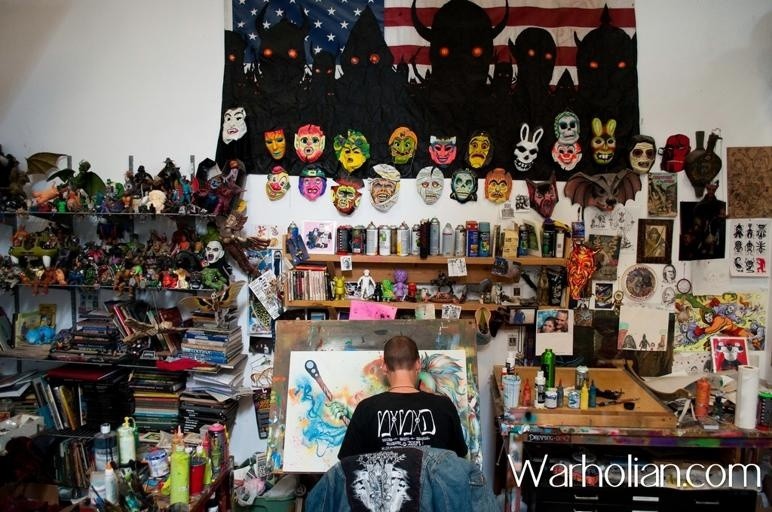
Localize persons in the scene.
[1,158,248,297]
[333,272,345,300]
[357,269,376,300]
[337,336,468,461]
[537,310,568,333]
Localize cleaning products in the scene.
[93,424,118,471]
[118,417,139,466]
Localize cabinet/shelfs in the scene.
[1,154,219,512]
[283,252,572,316]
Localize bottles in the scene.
[519,224,531,258]
[89,410,226,504]
[504,349,518,375]
[694,375,713,420]
[336,216,492,258]
[520,345,598,411]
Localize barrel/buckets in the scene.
[235,475,298,512]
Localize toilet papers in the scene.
[736,365,759,430]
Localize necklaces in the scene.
[388,385,416,390]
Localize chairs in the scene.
[192,464,236,512]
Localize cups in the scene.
[501,374,522,409]
